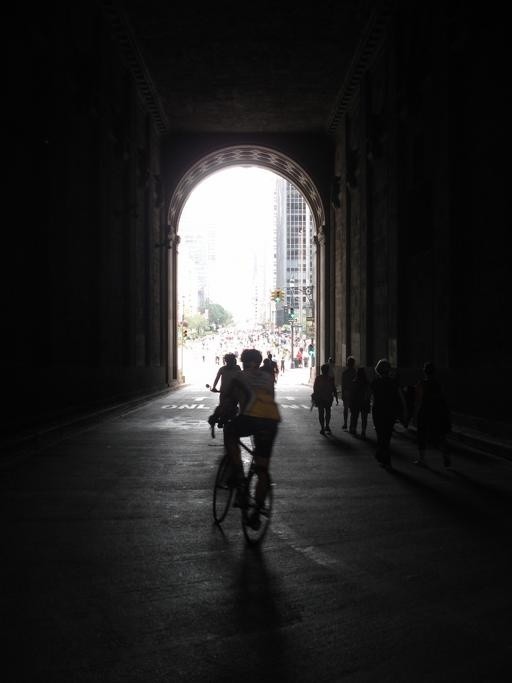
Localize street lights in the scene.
[288,274,297,368]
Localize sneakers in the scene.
[341,425,366,441]
[320,428,331,435]
[244,515,262,531]
[227,472,244,487]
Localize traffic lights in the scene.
[270,289,285,301]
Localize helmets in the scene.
[242,350,262,362]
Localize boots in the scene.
[374,453,399,474]
[412,451,424,466]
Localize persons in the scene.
[341,356,368,439]
[213,353,243,407]
[313,364,338,434]
[192,327,316,374]
[225,350,282,508]
[412,362,452,466]
[370,358,409,470]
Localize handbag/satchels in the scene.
[311,392,319,407]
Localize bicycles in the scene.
[211,412,273,546]
